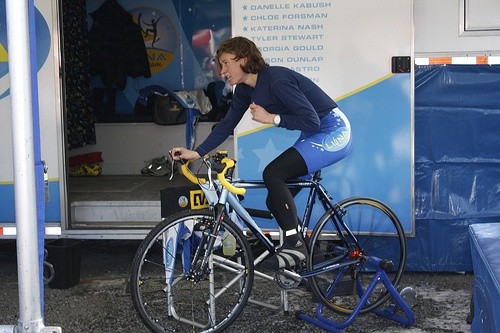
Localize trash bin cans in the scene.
[45,238,84,288]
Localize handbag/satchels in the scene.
[154,89,212,126]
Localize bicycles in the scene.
[130,151,407,333]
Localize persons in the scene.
[171,35,352,270]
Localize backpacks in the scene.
[207,81,234,119]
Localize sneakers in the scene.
[261,232,308,270]
[141,156,179,177]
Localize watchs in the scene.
[273,114,281,127]
